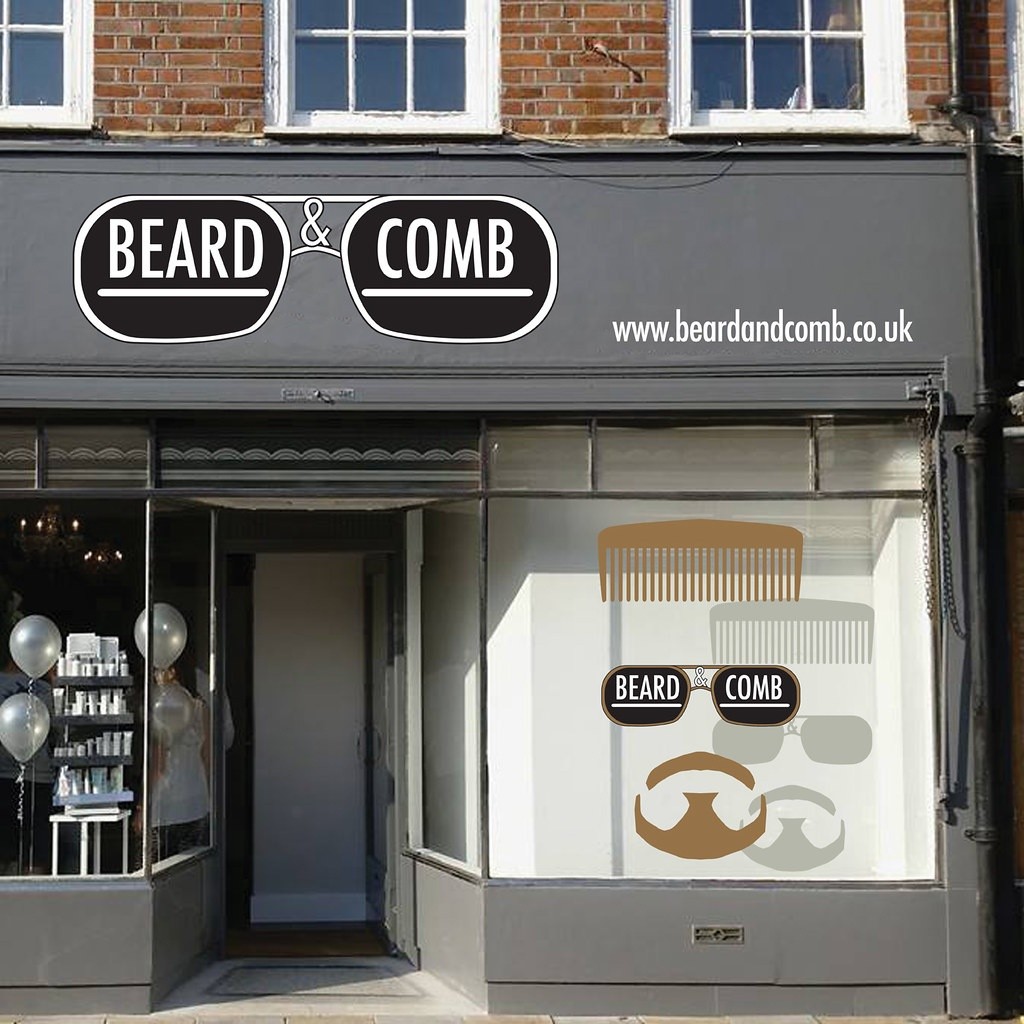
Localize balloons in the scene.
[151,683,195,729]
[135,602,188,670]
[8,614,62,681]
[0,692,50,763]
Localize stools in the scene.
[49,810,131,875]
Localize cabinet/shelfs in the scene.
[50,676,134,807]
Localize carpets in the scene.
[222,925,388,957]
